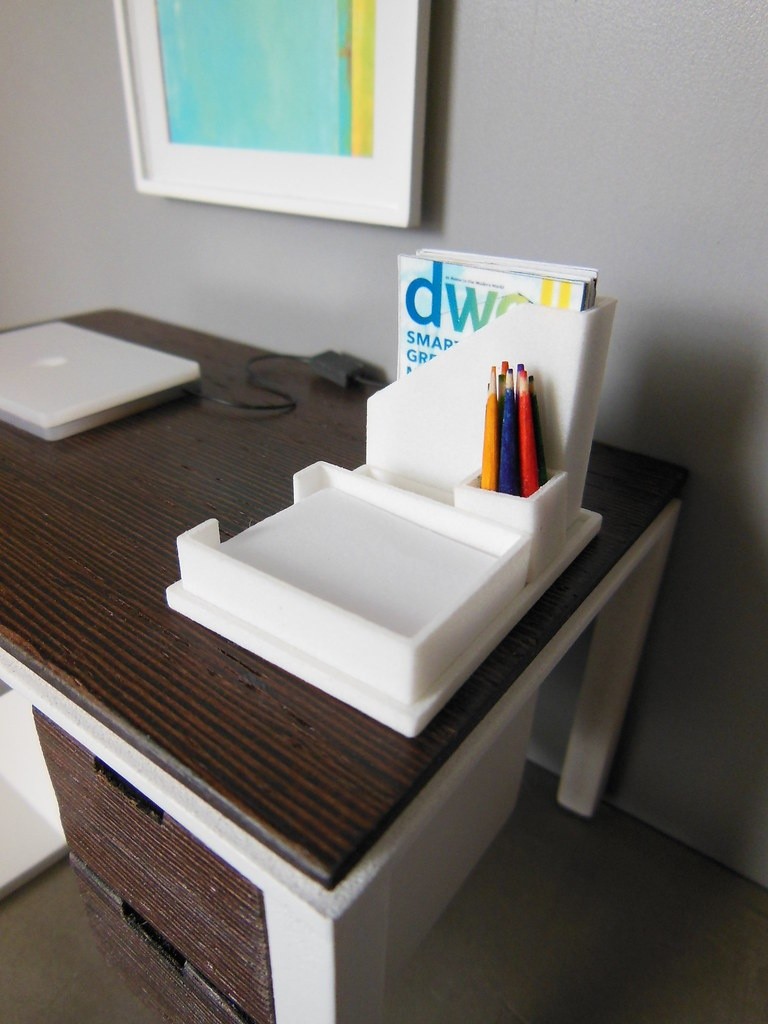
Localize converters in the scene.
[310,350,368,387]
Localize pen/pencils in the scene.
[478,356,551,512]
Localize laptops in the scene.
[0,320,201,441]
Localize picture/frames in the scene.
[115,0,429,233]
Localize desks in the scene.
[0,309,677,1024]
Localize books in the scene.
[395,243,602,380]
